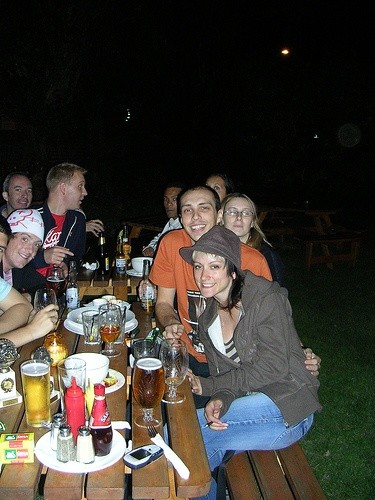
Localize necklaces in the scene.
[218,305,229,330]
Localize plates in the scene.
[62,367,125,394]
[35,426,126,473]
[63,300,138,336]
[126,269,143,277]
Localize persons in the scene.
[0,162,104,350]
[140,172,324,500]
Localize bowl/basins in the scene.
[132,257,153,273]
[64,353,110,384]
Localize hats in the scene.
[7,208,45,246]
[179,225,243,272]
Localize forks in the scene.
[147,424,158,438]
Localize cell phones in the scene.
[123,444,164,469]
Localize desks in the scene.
[0,302,211,500]
[78,223,156,301]
[273,205,336,271]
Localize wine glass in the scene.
[98,303,122,357]
[160,338,189,404]
[133,357,162,428]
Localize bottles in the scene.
[140,260,155,314]
[116,235,128,279]
[50,377,113,464]
[95,228,110,275]
[121,224,131,270]
[65,260,80,314]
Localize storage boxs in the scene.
[0,390,22,408]
[0,433,35,464]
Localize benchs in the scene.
[224,442,327,500]
[262,226,368,273]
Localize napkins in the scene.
[83,262,97,270]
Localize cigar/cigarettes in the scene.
[205,421,213,428]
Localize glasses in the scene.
[224,209,253,217]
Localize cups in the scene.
[57,358,87,421]
[134,339,159,359]
[46,268,66,309]
[82,295,127,345]
[34,288,55,332]
[21,332,68,427]
